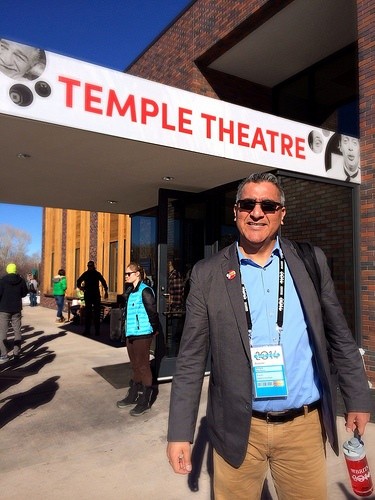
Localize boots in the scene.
[130,386,154,416]
[116,384,144,408]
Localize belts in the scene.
[251,398,321,424]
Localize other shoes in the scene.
[31,302,37,306]
[13,350,20,356]
[0,354,9,361]
[56,317,67,323]
[81,329,100,336]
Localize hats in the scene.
[6,263,17,274]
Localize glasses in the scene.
[236,199,283,211]
[124,272,136,276]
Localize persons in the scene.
[117,262,161,416]
[26,273,39,307]
[166,171,370,500]
[0,262,28,360]
[0,38,46,81]
[70,258,193,359]
[309,130,323,154]
[326,133,360,185]
[52,269,67,322]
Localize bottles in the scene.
[342,438,373,496]
